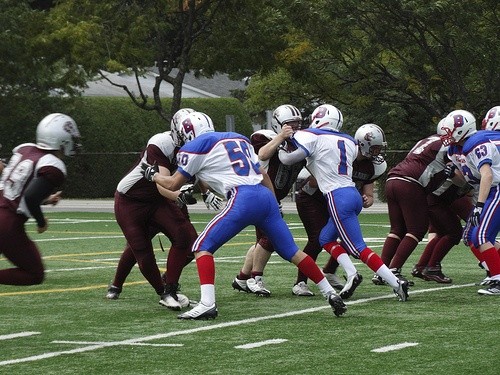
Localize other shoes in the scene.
[106,285,122,300]
[160,271,182,293]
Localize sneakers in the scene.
[421,263,454,283]
[159,294,182,310]
[326,292,348,317]
[176,293,191,308]
[339,274,363,299]
[395,279,409,301]
[477,280,500,295]
[292,281,316,297]
[320,269,344,289]
[245,276,270,296]
[176,302,219,321]
[377,267,414,285]
[411,264,429,281]
[371,272,384,285]
[476,275,491,286]
[232,273,249,292]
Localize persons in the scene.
[140,112,348,320]
[0,112,83,286]
[291,122,388,297]
[276,103,415,302]
[371,105,500,296]
[232,103,303,297]
[105,108,223,311]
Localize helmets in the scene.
[436,105,500,147]
[308,104,344,133]
[354,123,388,165]
[36,113,84,158]
[171,108,215,146]
[271,104,304,140]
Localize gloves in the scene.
[140,160,160,184]
[446,165,456,177]
[177,190,200,206]
[202,191,225,212]
[470,202,485,228]
